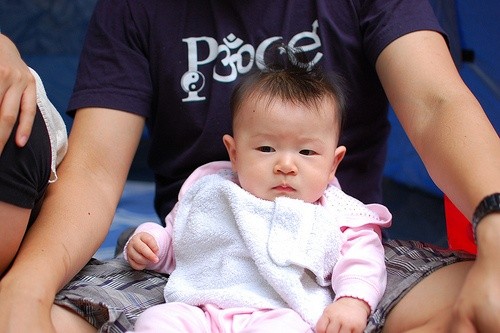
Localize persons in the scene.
[123,39,394,333]
[0,1,500,333]
[0,29,53,274]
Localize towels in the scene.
[163,174,344,333]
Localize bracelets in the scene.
[471,192,500,241]
